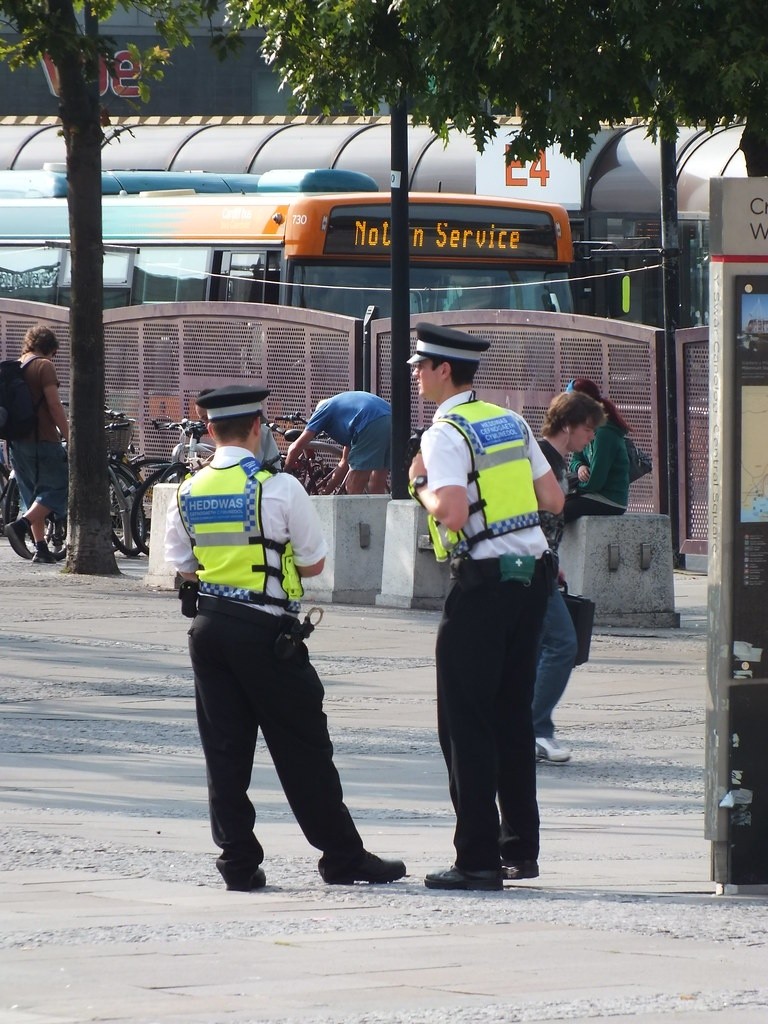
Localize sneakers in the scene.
[534,736,572,766]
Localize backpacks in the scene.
[0,355,52,442]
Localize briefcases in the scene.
[558,580,595,666]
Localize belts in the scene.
[197,595,302,631]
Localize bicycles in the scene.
[0,400,424,562]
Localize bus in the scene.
[1,189,637,531]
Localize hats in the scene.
[404,322,492,365]
[194,384,272,424]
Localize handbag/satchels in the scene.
[623,433,652,484]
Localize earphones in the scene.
[566,426,569,432]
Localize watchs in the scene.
[412,475,427,488]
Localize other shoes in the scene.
[318,850,407,885]
[500,856,540,880]
[31,551,57,564]
[2,521,34,559]
[423,864,504,891]
[226,867,267,892]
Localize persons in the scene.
[163,385,408,891]
[195,390,281,471]
[283,390,393,494]
[526,390,609,766]
[565,378,630,520]
[406,320,566,896]
[4,326,70,566]
